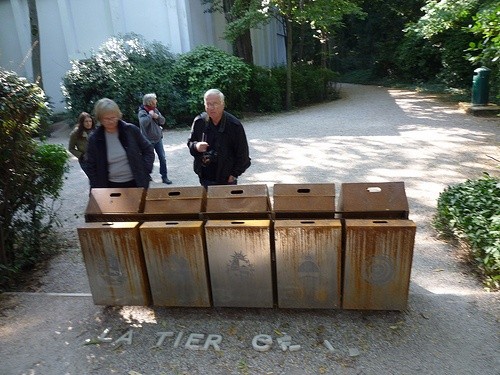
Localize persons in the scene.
[138,93,172,184]
[68,98,155,198]
[187,89,251,191]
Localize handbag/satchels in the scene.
[159,125,163,139]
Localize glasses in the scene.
[102,117,119,123]
[204,101,222,109]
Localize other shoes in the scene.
[161,175,172,184]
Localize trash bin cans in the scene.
[471,66,492,106]
